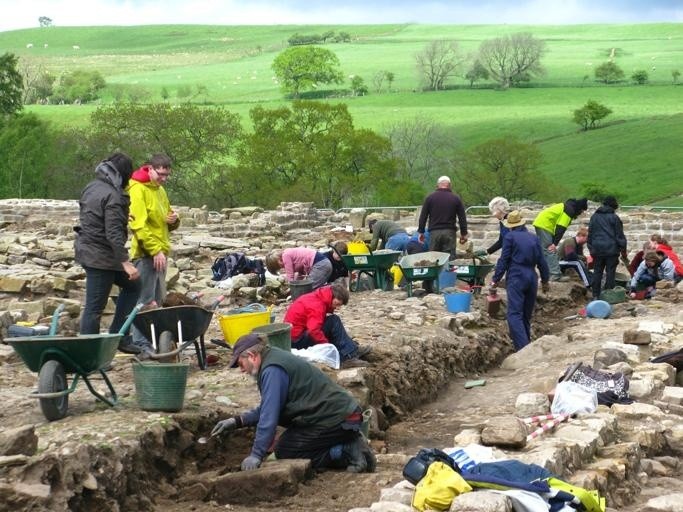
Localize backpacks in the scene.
[210,252,253,280]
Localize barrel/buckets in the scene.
[214,302,276,348]
[586,300,611,318]
[290,280,314,303]
[359,406,371,438]
[250,314,293,353]
[445,290,475,314]
[129,356,191,412]
[438,271,458,291]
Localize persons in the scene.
[210,333,377,473]
[73,153,142,354]
[557,228,593,292]
[283,283,372,368]
[491,210,549,351]
[369,176,468,290]
[628,234,683,300]
[532,198,587,282]
[485,197,514,256]
[266,246,332,296]
[588,196,627,301]
[127,155,180,351]
[324,241,348,285]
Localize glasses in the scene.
[154,169,169,177]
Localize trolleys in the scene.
[122,290,228,374]
[337,237,403,294]
[392,247,455,298]
[1,300,149,423]
[448,245,498,296]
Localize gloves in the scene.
[240,455,261,472]
[210,416,239,444]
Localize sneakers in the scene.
[357,430,377,474]
[352,345,369,358]
[117,334,142,354]
[343,357,368,369]
[343,436,370,473]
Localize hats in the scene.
[577,198,587,210]
[368,219,377,234]
[502,209,527,229]
[227,333,263,369]
[603,195,618,210]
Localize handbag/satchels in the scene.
[402,447,463,486]
[244,259,266,286]
[558,361,634,404]
